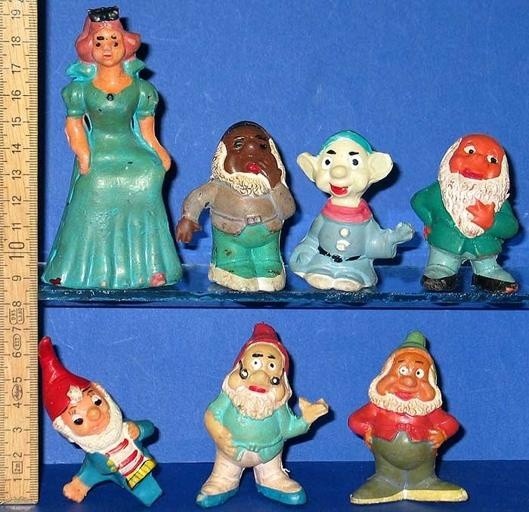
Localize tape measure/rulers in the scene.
[0,1,41,505]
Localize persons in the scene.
[39,335,165,507]
[194,322,331,509]
[174,119,297,293]
[287,129,414,294]
[40,5,184,290]
[409,133,520,294]
[345,330,469,505]
